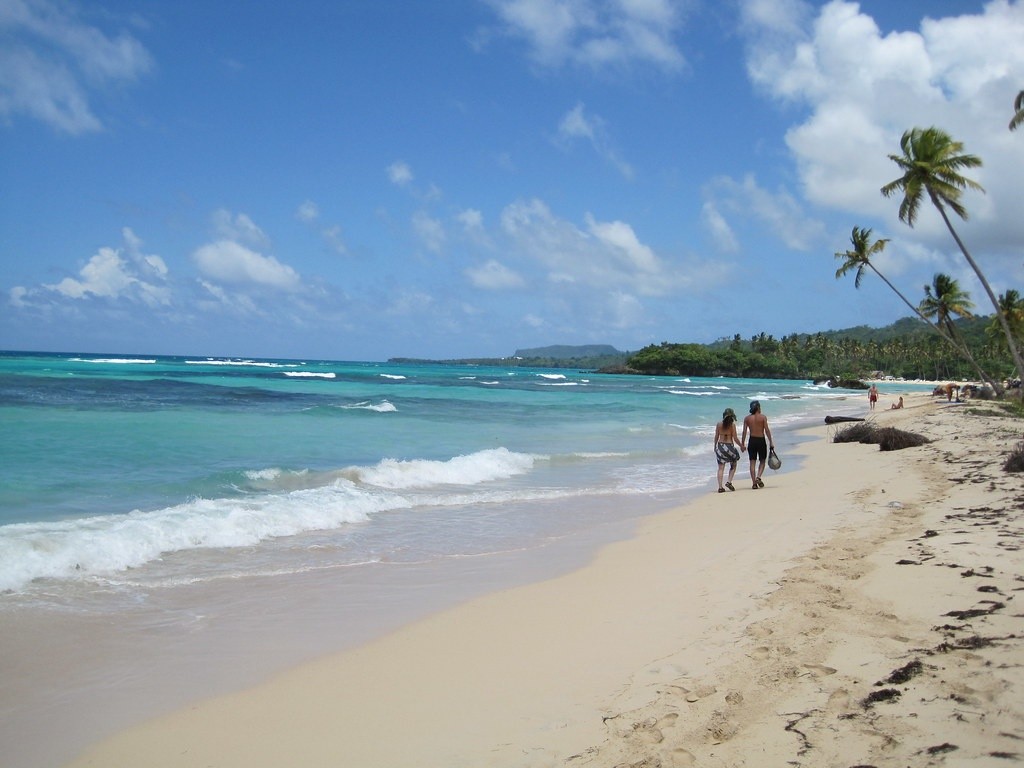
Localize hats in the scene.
[723,408,737,421]
[750,400,759,413]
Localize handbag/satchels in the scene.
[768,447,782,470]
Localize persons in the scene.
[891,397,903,409]
[867,383,879,410]
[933,377,1022,398]
[946,383,960,401]
[739,400,775,489]
[715,407,748,493]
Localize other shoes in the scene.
[752,484,758,489]
[725,482,735,491]
[755,477,764,487]
[718,488,725,493]
